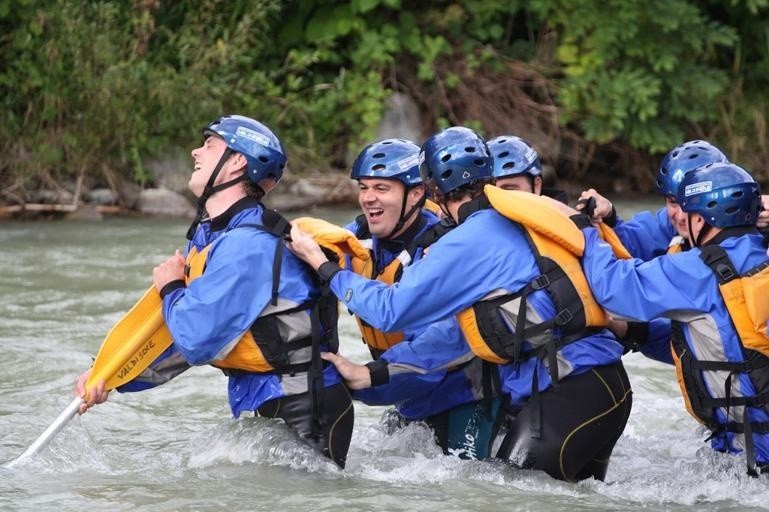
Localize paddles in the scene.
[9,273,191,465]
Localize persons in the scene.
[541,163,768,480]
[78,113,355,470]
[577,138,768,454]
[285,127,632,483]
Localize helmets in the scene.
[675,162,762,229]
[656,138,729,196]
[418,125,495,195]
[350,136,422,189]
[484,135,542,179]
[202,115,288,194]
